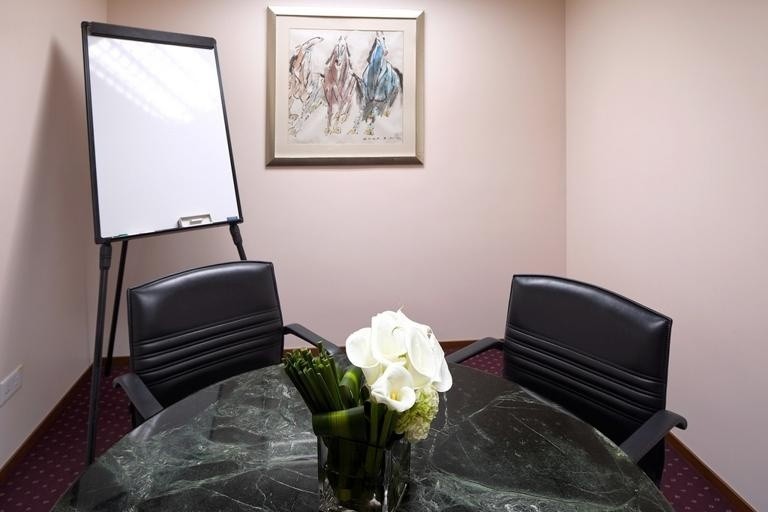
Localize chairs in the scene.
[113,262,339,428]
[444,274,687,490]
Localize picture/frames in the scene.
[265,5,424,168]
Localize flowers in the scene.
[284,309,453,442]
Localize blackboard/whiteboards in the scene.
[81,21,243,244]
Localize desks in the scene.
[50,362,676,510]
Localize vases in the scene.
[316,434,411,512]
